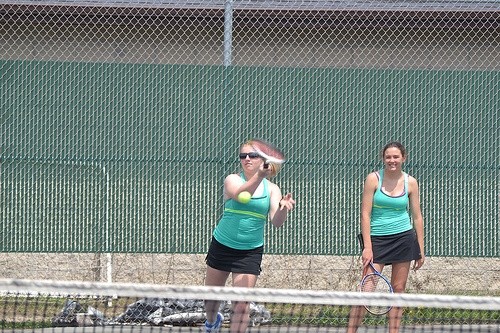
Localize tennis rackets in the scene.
[248,138,290,173]
[359,233,396,316]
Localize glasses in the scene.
[239,152,261,158]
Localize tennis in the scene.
[238,191,252,203]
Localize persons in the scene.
[204,139,296,333]
[347,141,425,333]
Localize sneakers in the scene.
[205,312,224,333]
